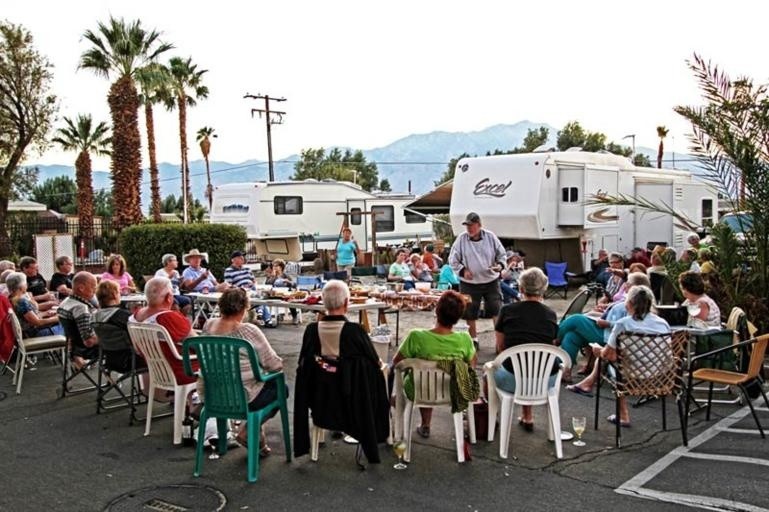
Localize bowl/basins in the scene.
[388,283,404,292]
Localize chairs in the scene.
[0,294,73,386]
[482,343,571,459]
[559,288,591,322]
[594,331,688,448]
[394,358,477,463]
[542,260,566,299]
[89,321,174,426]
[690,307,752,404]
[324,270,347,282]
[126,321,232,445]
[57,308,140,404]
[182,335,292,483]
[309,359,390,462]
[7,307,73,395]
[683,334,769,439]
[295,276,323,324]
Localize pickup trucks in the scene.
[698,211,769,271]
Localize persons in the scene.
[387,292,478,438]
[154,254,191,318]
[584,254,628,318]
[682,235,716,292]
[301,279,383,441]
[482,267,559,433]
[584,248,610,308]
[223,251,277,328]
[183,249,228,326]
[200,260,220,286]
[439,255,461,292]
[564,284,671,427]
[386,243,443,291]
[89,280,175,405]
[56,271,100,360]
[1,270,58,313]
[101,255,136,295]
[499,280,525,306]
[577,263,647,375]
[265,258,299,325]
[0,260,55,301]
[202,285,289,456]
[48,256,73,301]
[501,250,525,288]
[259,262,273,286]
[19,256,55,303]
[335,229,360,287]
[128,276,203,428]
[625,244,676,277]
[553,272,651,384]
[449,213,507,356]
[676,271,720,403]
[6,272,92,369]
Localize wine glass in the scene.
[232,419,241,429]
[208,433,219,459]
[393,438,407,469]
[572,416,586,446]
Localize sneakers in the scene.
[518,416,534,432]
[608,414,631,426]
[566,384,595,398]
[416,424,432,438]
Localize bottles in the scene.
[181,404,193,448]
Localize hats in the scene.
[232,251,247,259]
[506,251,520,259]
[184,249,205,263]
[463,213,480,226]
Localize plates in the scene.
[344,435,359,442]
[560,430,574,439]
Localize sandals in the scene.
[239,427,274,458]
